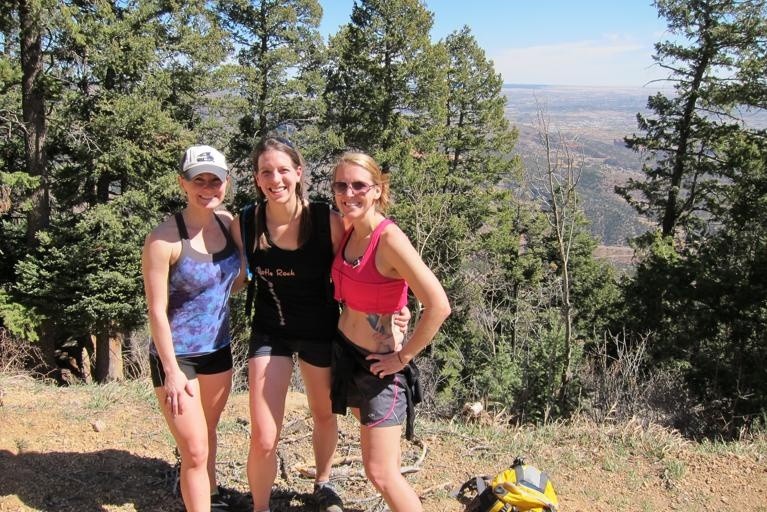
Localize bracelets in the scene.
[396,351,409,366]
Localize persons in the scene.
[330,151,452,512]
[140,143,236,512]
[229,135,347,512]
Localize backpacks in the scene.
[460,456,561,512]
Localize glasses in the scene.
[329,180,378,197]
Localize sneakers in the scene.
[211,485,253,512]
[313,482,345,512]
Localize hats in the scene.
[182,145,230,183]
